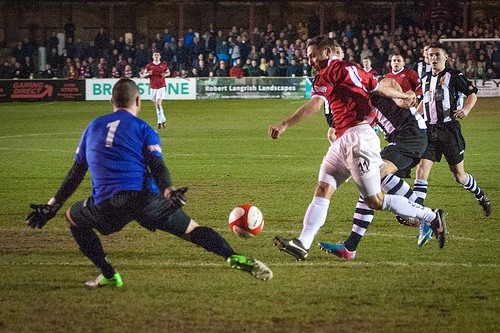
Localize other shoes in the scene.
[158,123,161,130]
[163,123,166,129]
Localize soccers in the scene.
[228,204,264,239]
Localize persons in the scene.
[1,0,500,288]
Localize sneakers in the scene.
[228,255,273,281]
[418,222,434,248]
[395,214,418,227]
[273,236,310,262]
[318,241,357,261]
[84,273,123,288]
[429,209,448,249]
[476,191,491,216]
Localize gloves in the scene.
[167,187,188,211]
[25,203,57,229]
